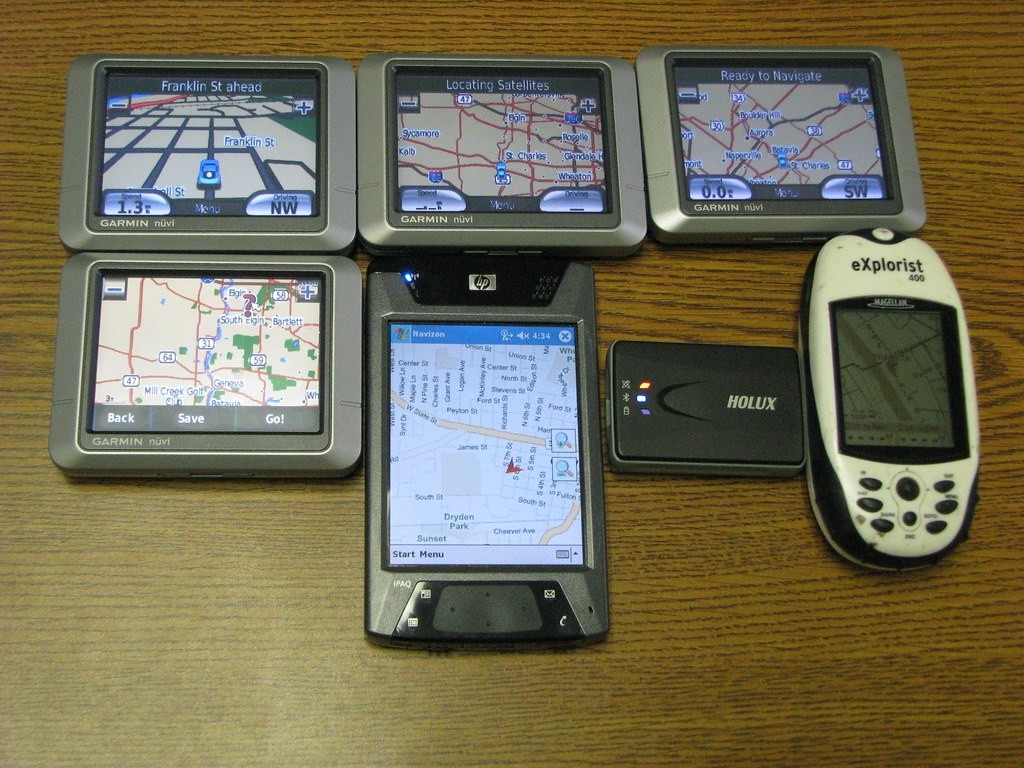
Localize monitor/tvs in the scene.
[51,44,928,650]
